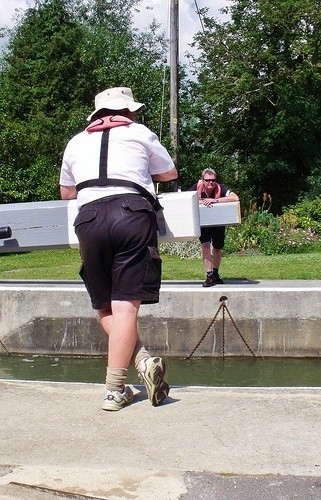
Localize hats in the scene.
[86,87,146,123]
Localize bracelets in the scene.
[216,198,218,202]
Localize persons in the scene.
[189,168,240,287]
[59,87,178,411]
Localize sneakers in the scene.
[203,275,215,286]
[102,386,133,411]
[138,357,170,407]
[213,274,224,283]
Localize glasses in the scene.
[203,179,216,182]
